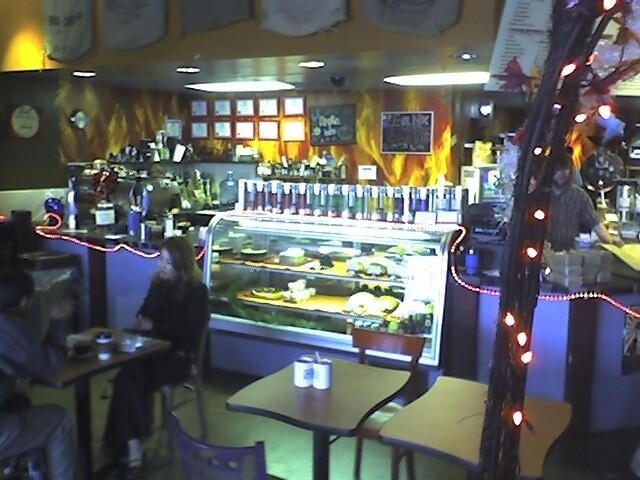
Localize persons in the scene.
[98,236,213,480]
[0,265,77,480]
[544,148,625,249]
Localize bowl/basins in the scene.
[240,250,267,261]
[279,252,305,266]
[212,247,234,258]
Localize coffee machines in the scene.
[466,199,512,283]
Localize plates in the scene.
[67,350,97,360]
[249,289,284,300]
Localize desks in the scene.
[30,327,173,480]
[225,355,413,480]
[379,375,584,480]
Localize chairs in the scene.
[172,418,285,480]
[152,312,210,466]
[350,328,425,480]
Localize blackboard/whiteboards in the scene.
[380,111,434,154]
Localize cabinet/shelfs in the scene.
[203,211,458,406]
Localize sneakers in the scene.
[126,450,146,479]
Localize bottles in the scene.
[128,209,140,236]
[463,246,480,277]
[218,170,238,211]
[240,179,469,226]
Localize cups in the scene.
[293,356,314,388]
[92,331,114,362]
[312,359,332,391]
[72,338,90,355]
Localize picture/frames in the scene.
[188,95,306,143]
[164,119,182,140]
